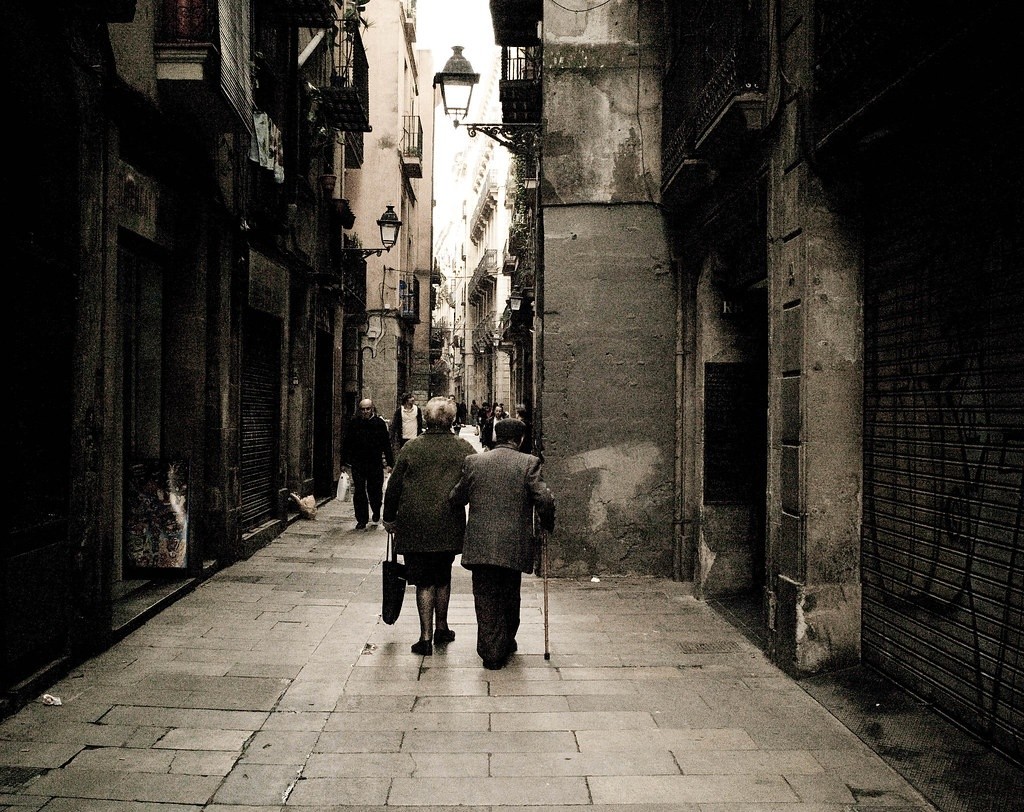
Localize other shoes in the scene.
[434,630,456,643]
[484,661,503,669]
[411,641,432,656]
[509,641,517,651]
[356,520,367,528]
[372,511,381,522]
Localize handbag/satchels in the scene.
[337,471,352,502]
[382,528,407,625]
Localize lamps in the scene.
[376,205,402,252]
[432,45,482,129]
[509,285,524,311]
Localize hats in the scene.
[495,419,526,437]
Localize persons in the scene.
[389,392,423,455]
[446,419,557,669]
[432,390,532,453]
[383,397,479,655]
[340,398,393,529]
